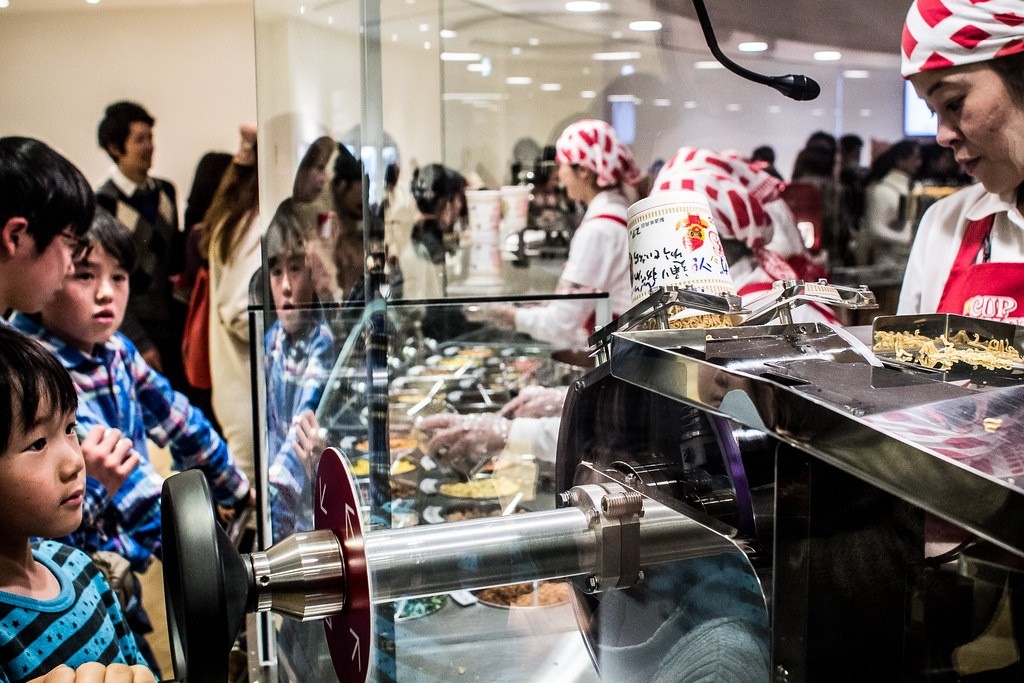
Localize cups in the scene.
[468,232,503,281]
[465,190,504,245]
[627,191,747,329]
[499,186,530,232]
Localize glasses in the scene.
[57,230,91,263]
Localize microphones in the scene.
[692,0,820,101]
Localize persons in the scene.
[0,135,141,552]
[467,118,640,346]
[0,324,166,683]
[9,202,255,683]
[892,0,1024,326]
[415,144,844,465]
[636,118,975,329]
[93,100,180,373]
[186,120,562,555]
[255,133,380,683]
[686,358,775,438]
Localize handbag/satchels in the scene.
[182,269,212,388]
